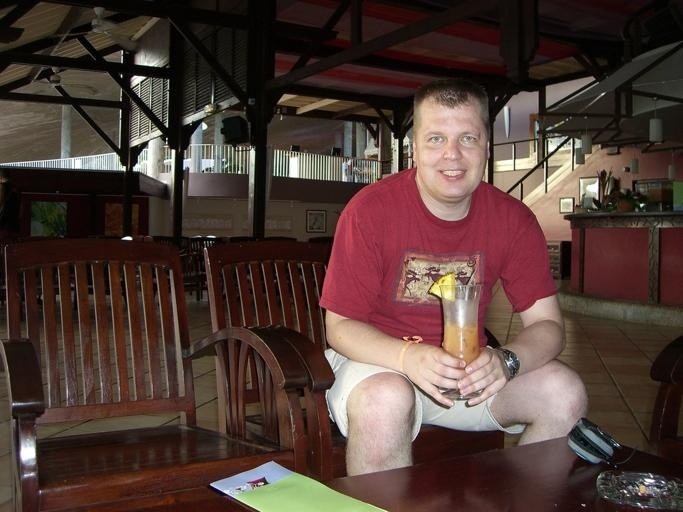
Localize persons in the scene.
[318,75,589,478]
[341,155,378,184]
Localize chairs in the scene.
[2,235,334,316]
[2,234,307,512]
[201,240,504,481]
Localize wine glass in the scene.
[441,284,483,401]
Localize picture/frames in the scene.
[559,197,575,214]
[578,175,601,209]
[306,210,327,232]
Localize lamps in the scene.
[668,149,677,180]
[630,143,639,173]
[574,116,592,165]
[646,96,664,143]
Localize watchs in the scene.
[493,347,520,381]
